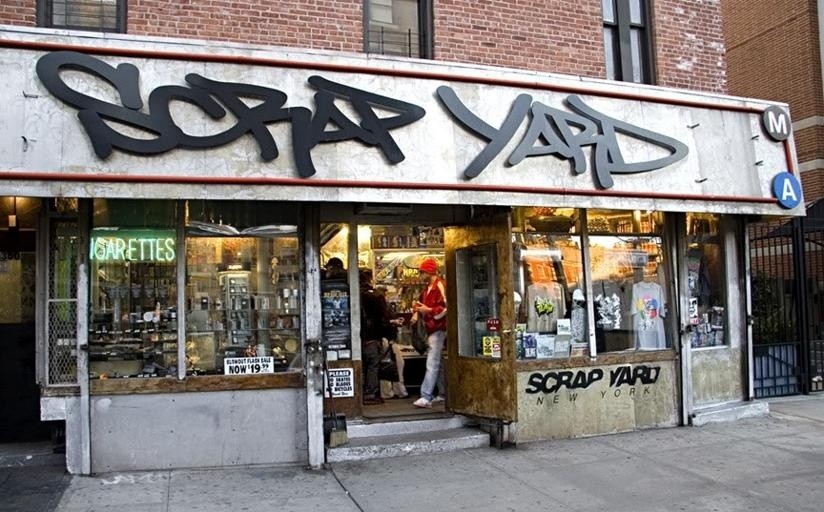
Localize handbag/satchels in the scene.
[411,319,429,355]
[379,360,399,382]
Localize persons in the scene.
[320,255,412,408]
[408,258,448,410]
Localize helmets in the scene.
[324,258,345,270]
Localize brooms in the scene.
[321,341,349,447]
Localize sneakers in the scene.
[432,395,445,403]
[413,397,432,409]
[364,393,410,404]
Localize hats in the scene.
[360,269,372,279]
[419,260,437,274]
